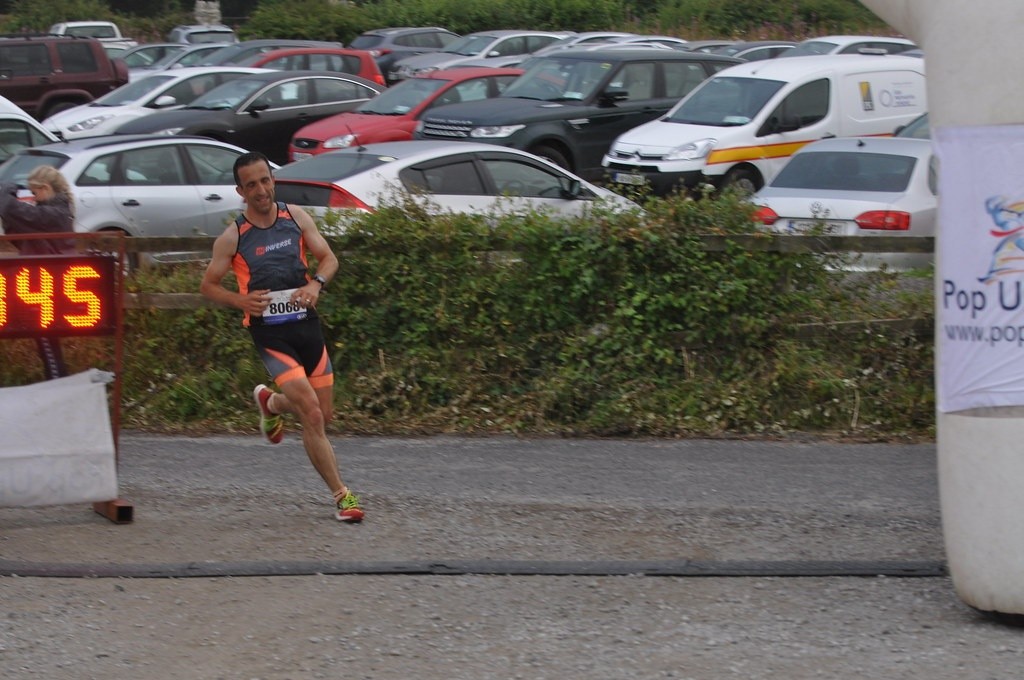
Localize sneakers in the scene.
[254,384,284,444]
[335,489,363,523]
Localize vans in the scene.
[600,53,926,202]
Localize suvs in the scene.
[415,47,751,178]
[0,33,128,123]
[0,132,279,277]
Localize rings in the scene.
[305,299,311,302]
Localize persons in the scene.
[0,166,82,254]
[201,153,367,523]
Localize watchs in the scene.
[312,275,326,287]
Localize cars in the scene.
[41,66,284,142]
[48,21,919,88]
[740,137,938,276]
[288,68,567,158]
[116,71,388,166]
[272,138,650,235]
